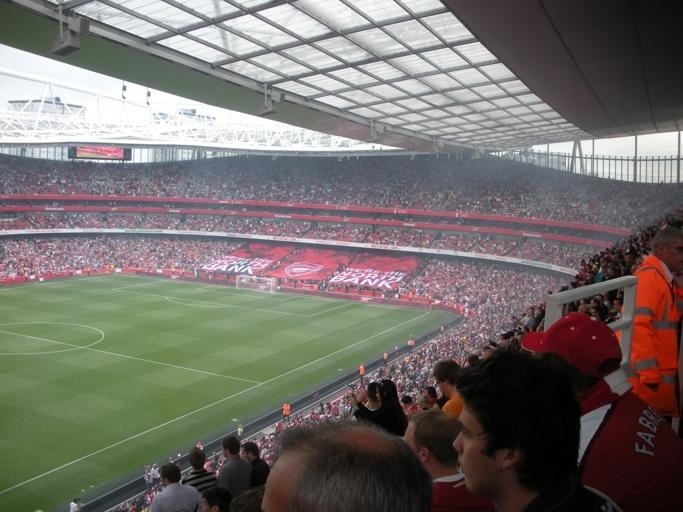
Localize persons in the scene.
[1,150,683,511]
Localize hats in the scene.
[520,311,621,380]
